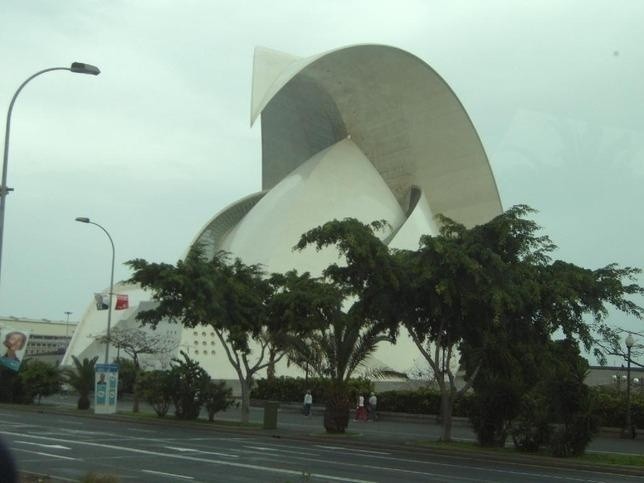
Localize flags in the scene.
[115,294,128,310]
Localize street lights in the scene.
[0,61,101,284]
[74,217,116,364]
[620,330,635,439]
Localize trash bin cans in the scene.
[264,401,279,429]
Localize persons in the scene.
[97,374,106,384]
[60,382,68,396]
[355,392,364,420]
[304,390,313,416]
[2,332,26,362]
[368,392,379,420]
[95,295,108,310]
